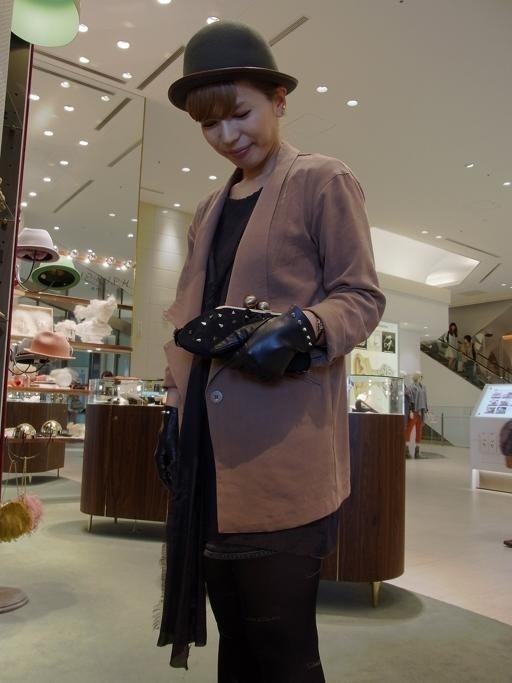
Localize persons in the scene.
[442,321,460,370]
[497,418,512,547]
[405,369,430,460]
[95,370,117,396]
[153,21,389,682]
[459,335,478,383]
[400,370,412,430]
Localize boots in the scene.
[415,446,423,458]
[405,446,412,459]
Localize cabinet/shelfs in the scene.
[79,374,412,607]
[2,402,68,478]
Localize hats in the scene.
[32,256,80,290]
[24,330,76,360]
[17,228,59,262]
[167,19,298,112]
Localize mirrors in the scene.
[0,49,148,401]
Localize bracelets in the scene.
[312,310,328,345]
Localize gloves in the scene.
[209,303,316,385]
[155,406,184,497]
